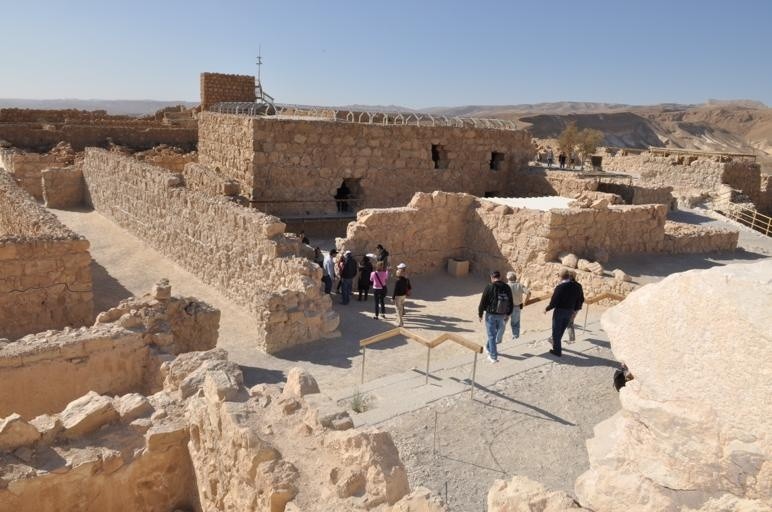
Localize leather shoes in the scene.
[325,290,404,327]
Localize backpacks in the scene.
[486,282,510,316]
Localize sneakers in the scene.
[484,336,575,363]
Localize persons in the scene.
[297,230,413,328]
[530,141,578,170]
[477,268,514,364]
[548,268,585,343]
[540,267,581,357]
[504,270,531,340]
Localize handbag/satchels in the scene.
[382,285,388,297]
[405,278,412,297]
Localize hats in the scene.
[330,249,339,255]
[345,251,351,256]
[396,263,409,269]
